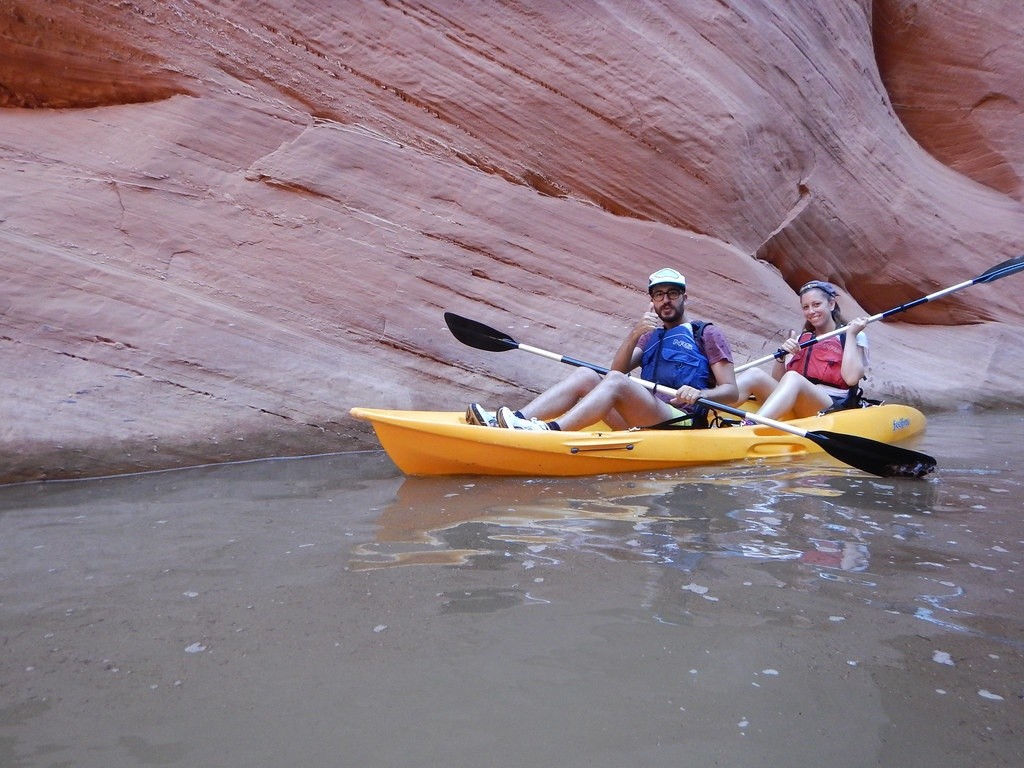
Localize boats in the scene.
[340,394,929,475]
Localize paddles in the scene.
[729,254,1024,374]
[442,310,940,480]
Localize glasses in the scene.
[650,289,684,302]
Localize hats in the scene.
[648,268,686,293]
[799,280,841,313]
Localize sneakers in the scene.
[496,405,551,431]
[720,418,758,428]
[465,402,501,428]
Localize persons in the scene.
[708,280,868,428]
[472,267,740,433]
